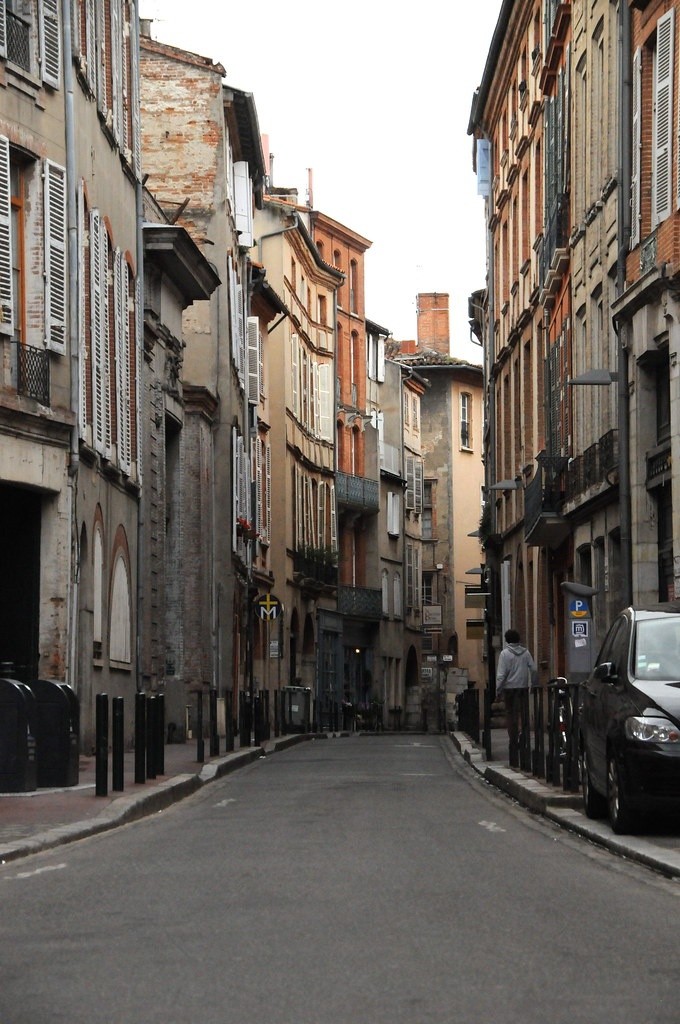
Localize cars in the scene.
[577,601,680,835]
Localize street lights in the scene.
[465,563,492,762]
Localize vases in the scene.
[236,524,244,537]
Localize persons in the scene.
[495,630,540,746]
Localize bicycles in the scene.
[548,677,573,764]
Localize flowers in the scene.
[236,515,250,529]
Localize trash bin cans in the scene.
[0,679,39,793]
[284,685,312,733]
[22,680,81,787]
